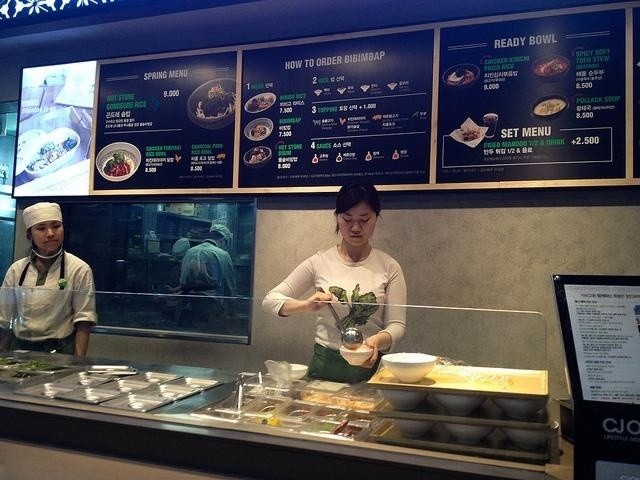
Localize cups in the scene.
[484,113,499,138]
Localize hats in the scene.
[22,202,65,232]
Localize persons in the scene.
[162,224,240,335]
[0,202,98,357]
[262,181,407,383]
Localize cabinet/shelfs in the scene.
[134,211,256,266]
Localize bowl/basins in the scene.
[530,95,570,122]
[240,92,277,168]
[290,363,308,381]
[96,141,142,183]
[532,55,572,83]
[381,351,438,384]
[15,128,80,181]
[340,346,373,366]
[442,63,480,91]
[186,76,237,131]
